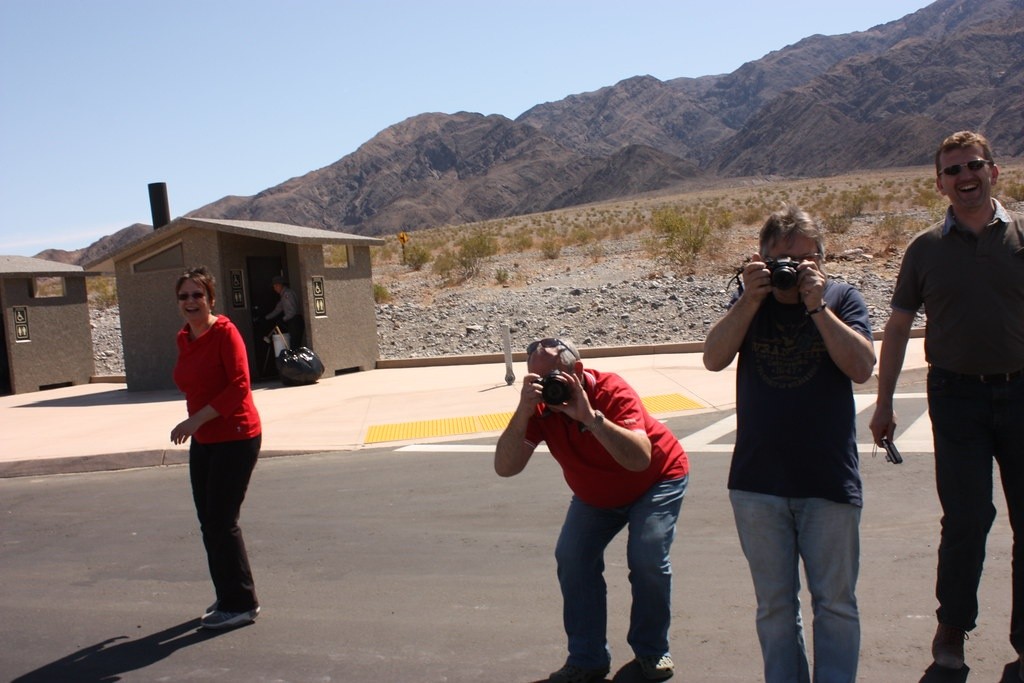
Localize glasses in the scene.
[178,292,207,301]
[527,338,577,361]
[938,159,992,175]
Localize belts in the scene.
[931,366,1024,385]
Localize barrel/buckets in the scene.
[273,333,290,357]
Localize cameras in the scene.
[765,257,804,289]
[531,369,571,406]
[881,438,903,464]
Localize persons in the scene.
[868,131,1023,668]
[170,265,261,627]
[704,206,877,683]
[265,279,305,350]
[494,336,689,683]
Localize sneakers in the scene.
[1019,653,1024,681]
[550,663,609,683]
[932,622,969,668]
[201,607,260,629]
[636,654,674,679]
[205,600,218,613]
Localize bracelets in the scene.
[585,409,604,430]
[805,302,826,315]
